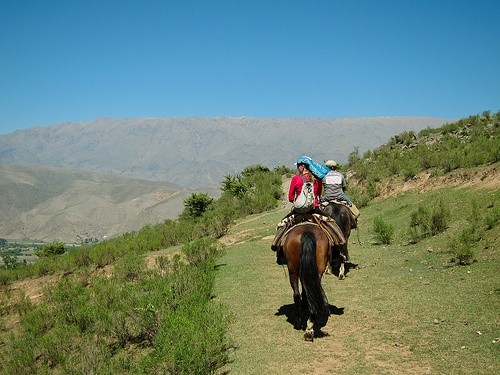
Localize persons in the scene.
[276,156,347,259]
[315,158,354,207]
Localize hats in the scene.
[326,160,337,167]
[294,156,329,179]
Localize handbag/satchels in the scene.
[344,203,361,220]
[270,214,298,251]
[315,213,346,245]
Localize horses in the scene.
[283,224,330,342]
[322,201,354,280]
